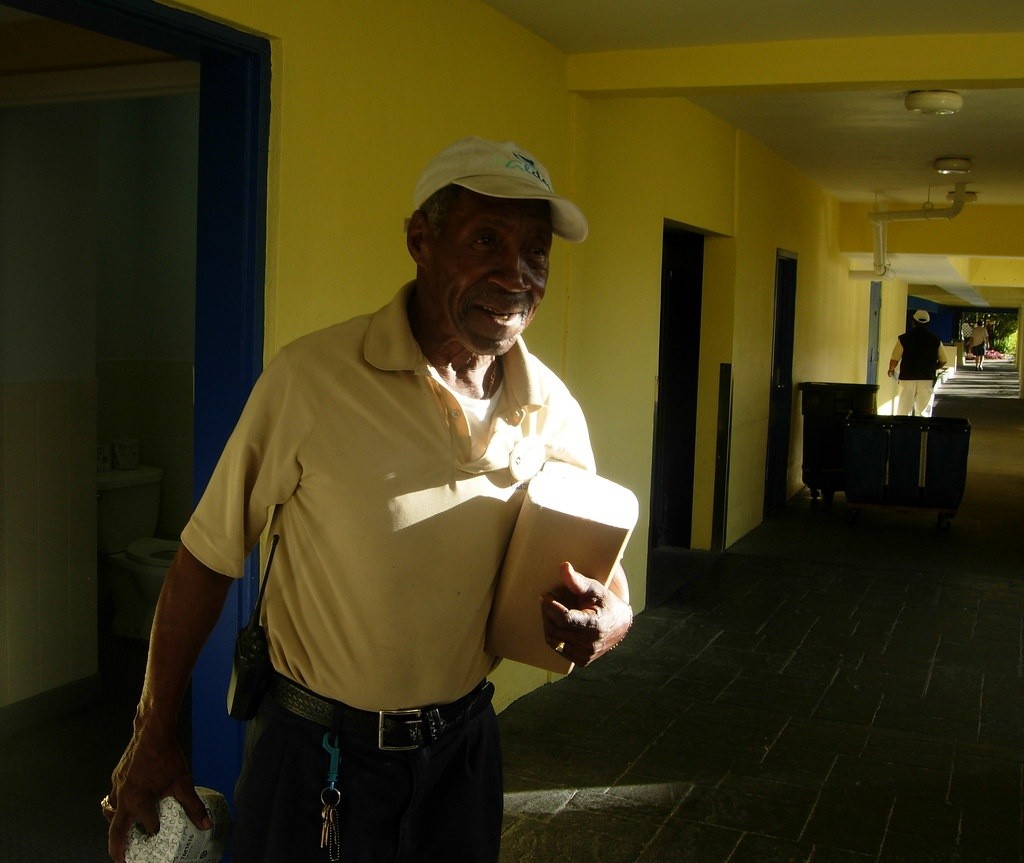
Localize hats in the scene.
[913,310,930,323]
[404,137,588,243]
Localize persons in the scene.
[108,138,634,863]
[972,321,990,371]
[888,309,949,417]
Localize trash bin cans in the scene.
[843,415,970,509]
[797,380,879,498]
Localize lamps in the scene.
[931,157,972,175]
[905,89,964,119]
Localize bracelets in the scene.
[889,369,894,372]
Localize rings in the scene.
[101,796,115,815]
[557,643,564,651]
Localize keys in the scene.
[320,802,339,850]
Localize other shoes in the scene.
[976,365,983,371]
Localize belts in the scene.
[265,670,494,755]
